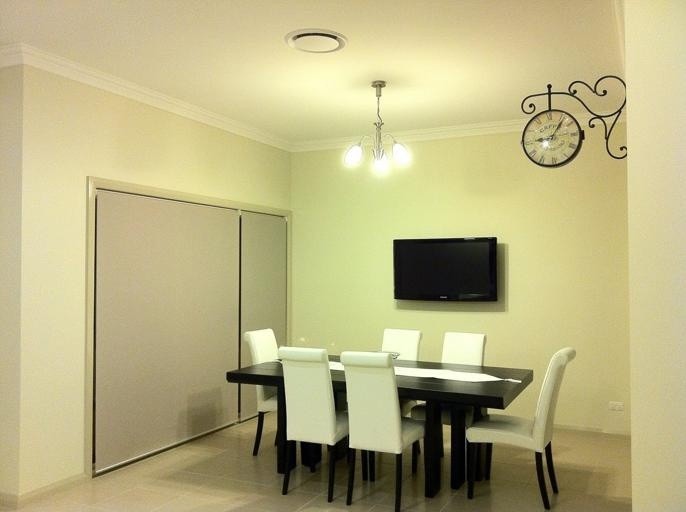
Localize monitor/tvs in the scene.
[394,237,498,301]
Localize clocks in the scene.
[520,109,585,168]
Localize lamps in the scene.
[341,80,411,175]
